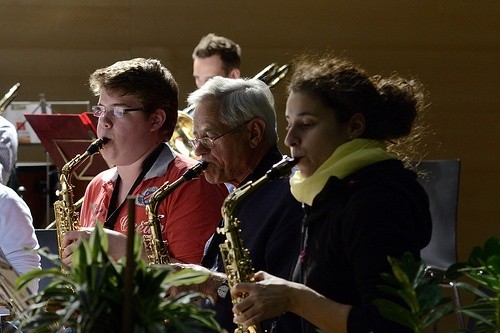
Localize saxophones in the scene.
[52,137,111,267]
[217,156,298,333]
[141,161,209,264]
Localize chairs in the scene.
[410,158,466,333]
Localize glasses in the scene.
[91,105,146,118]
[189,116,257,148]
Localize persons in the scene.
[0,182,42,333]
[60,56,230,274]
[163,75,303,333]
[0,115,18,186]
[230,49,434,333]
[166,33,242,194]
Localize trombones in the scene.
[177,63,292,140]
